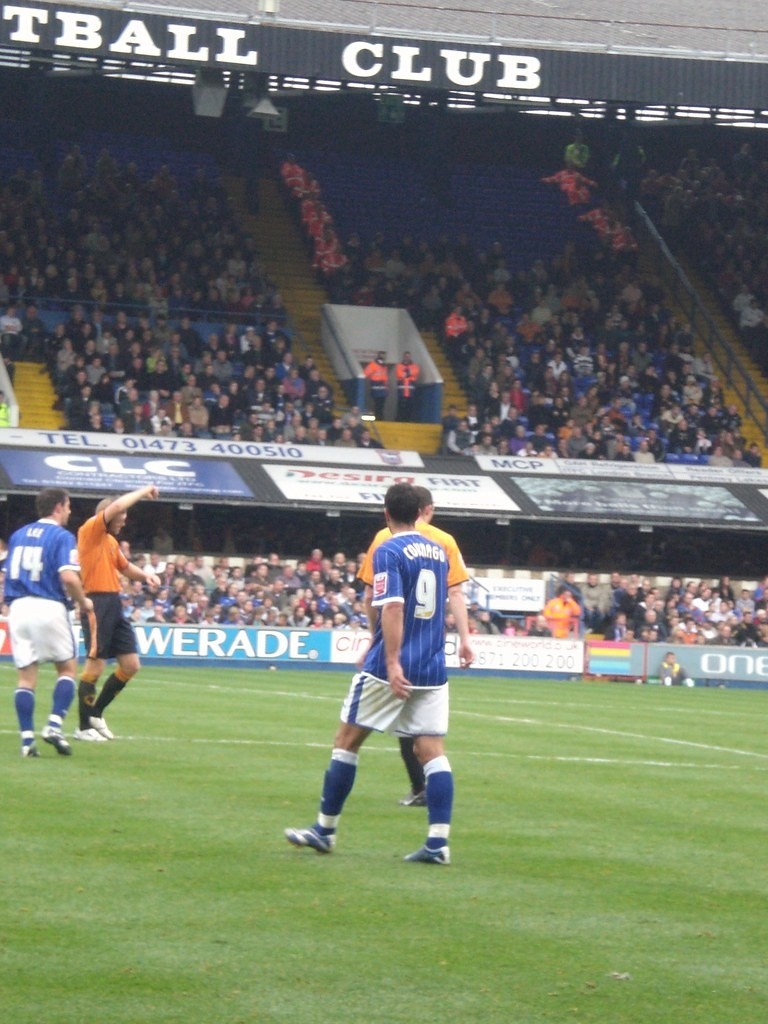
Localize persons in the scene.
[72,485,161,742]
[658,652,695,687]
[281,127,768,468]
[354,486,475,807]
[282,483,455,865]
[0,487,93,758]
[364,350,389,420]
[0,495,768,649]
[0,144,372,450]
[395,350,420,422]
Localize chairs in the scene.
[0,106,768,464]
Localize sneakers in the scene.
[73,728,107,743]
[89,716,113,740]
[405,846,450,866]
[42,726,72,755]
[399,790,428,807]
[23,744,38,757]
[285,827,336,854]
